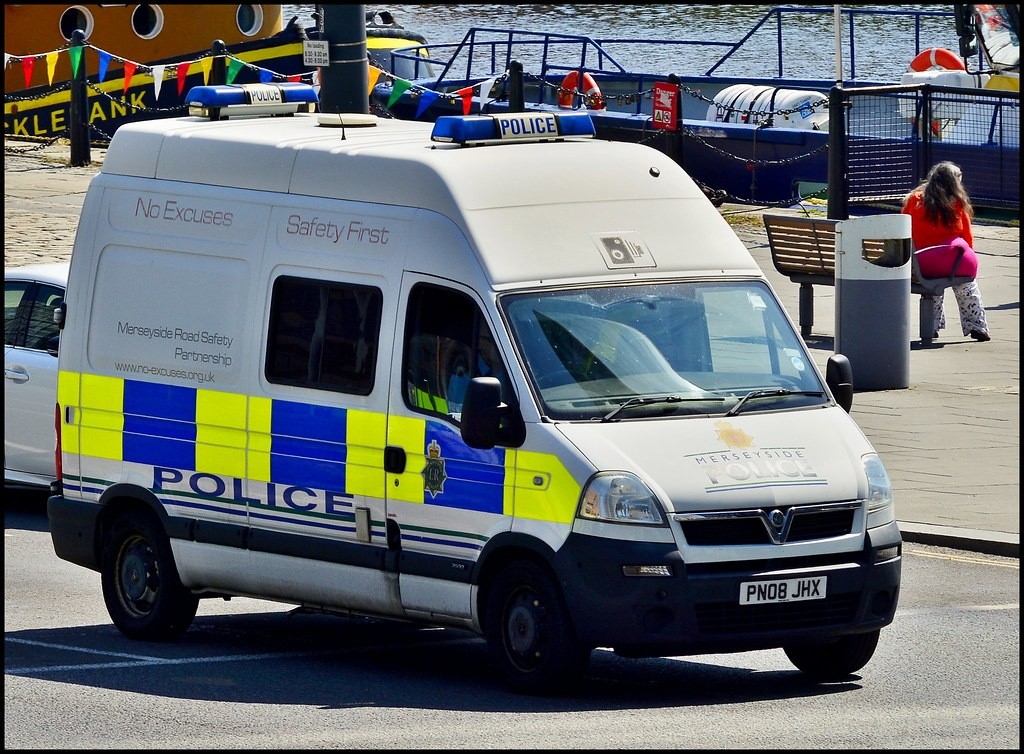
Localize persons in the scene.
[901,162,990,340]
[448,320,504,406]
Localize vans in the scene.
[51,86,904,701]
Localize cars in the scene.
[1,262,72,495]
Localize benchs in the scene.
[762,214,975,350]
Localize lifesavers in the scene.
[555,68,612,118]
[905,47,972,138]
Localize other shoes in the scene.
[971,330,992,343]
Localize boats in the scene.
[1,2,437,138]
[367,5,1024,214]
